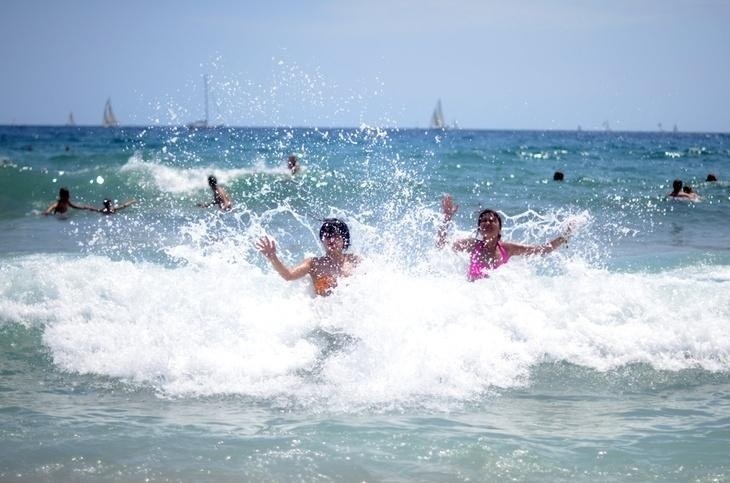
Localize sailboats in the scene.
[100,96,121,128]
[188,76,226,128]
[429,98,447,130]
[66,111,77,127]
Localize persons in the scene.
[199,174,233,211]
[705,173,718,182]
[553,170,564,180]
[42,186,90,220]
[287,152,300,175]
[681,184,703,202]
[84,198,136,213]
[666,178,693,198]
[253,216,362,300]
[435,193,578,283]
